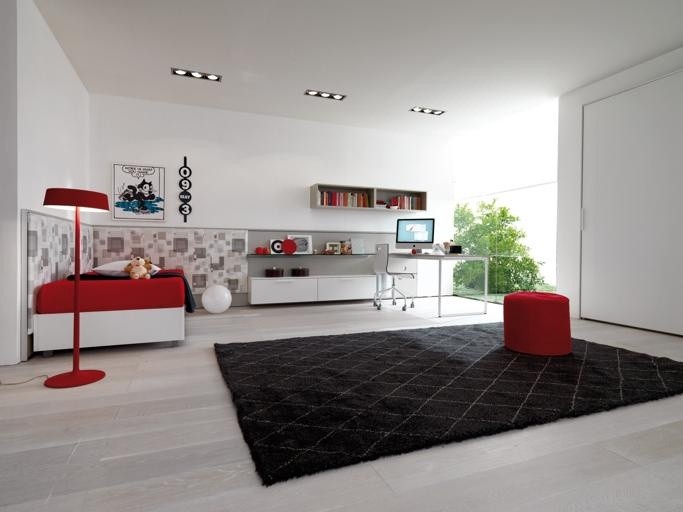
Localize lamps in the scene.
[43,188,110,388]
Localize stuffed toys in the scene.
[123,256,152,279]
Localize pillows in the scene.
[92,260,162,277]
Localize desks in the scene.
[390,252,490,317]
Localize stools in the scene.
[504,292,573,357]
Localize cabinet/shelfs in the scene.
[248,253,378,305]
[309,183,427,213]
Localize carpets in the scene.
[214,322,682,488]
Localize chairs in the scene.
[373,244,417,310]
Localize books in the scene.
[318,191,421,210]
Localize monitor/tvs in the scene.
[395,218,434,255]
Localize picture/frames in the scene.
[325,242,342,255]
[112,163,166,222]
[287,235,313,254]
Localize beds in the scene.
[33,269,185,358]
